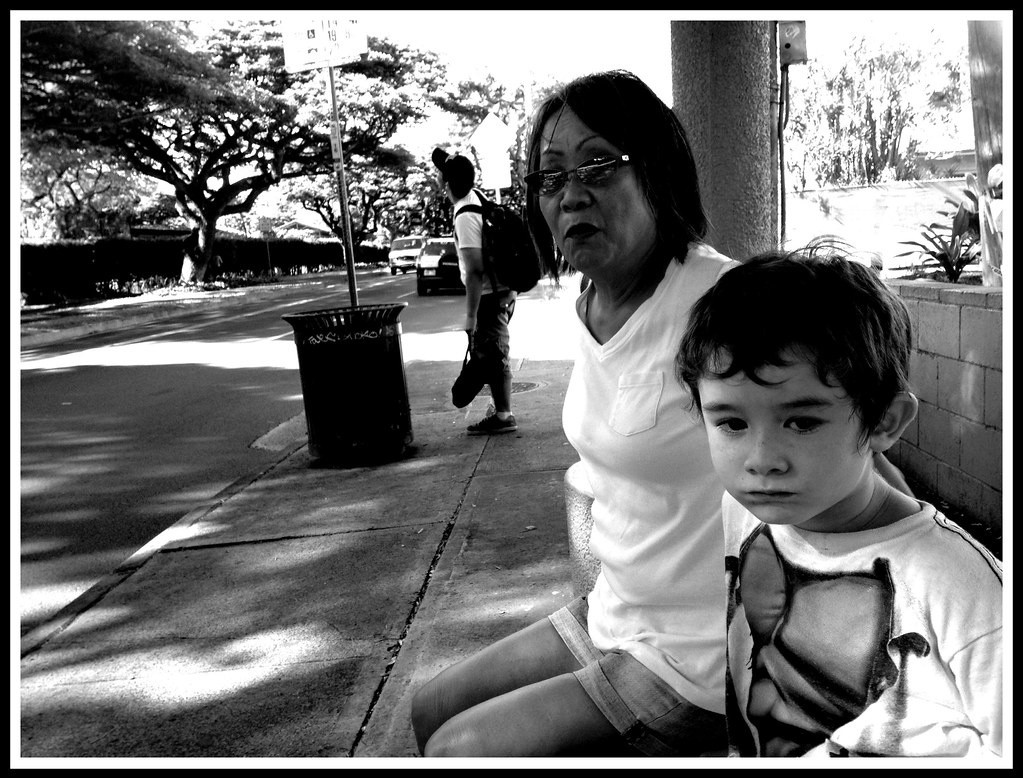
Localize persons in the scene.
[986,163,1002,240]
[411,73,915,757]
[683,250,1004,758]
[432,147,519,434]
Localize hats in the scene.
[432,146,476,188]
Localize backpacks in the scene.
[454,190,539,292]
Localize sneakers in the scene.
[466,412,518,436]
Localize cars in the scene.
[416,235,457,297]
[388,234,426,276]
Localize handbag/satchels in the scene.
[452,332,485,408]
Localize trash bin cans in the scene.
[280,301,414,459]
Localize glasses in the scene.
[524,154,630,198]
